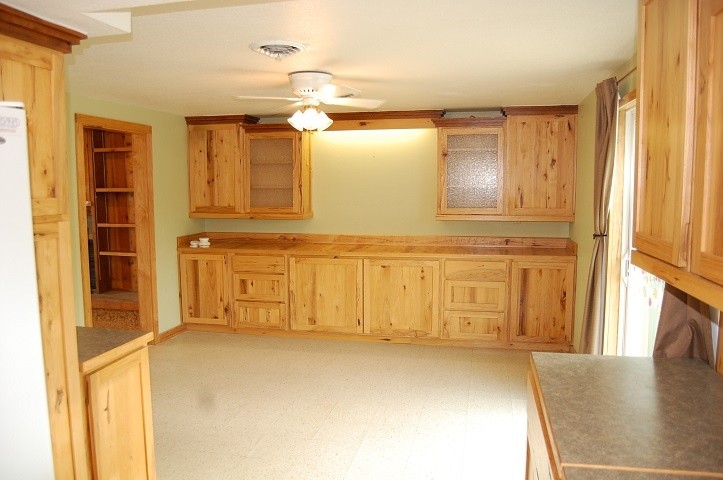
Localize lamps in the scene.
[287,98,333,133]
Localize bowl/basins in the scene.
[190,238,211,248]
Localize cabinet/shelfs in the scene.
[435,114,578,222]
[525,370,558,480]
[631,0,723,312]
[187,123,313,219]
[1,34,155,480]
[180,253,577,352]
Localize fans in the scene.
[238,72,385,113]
